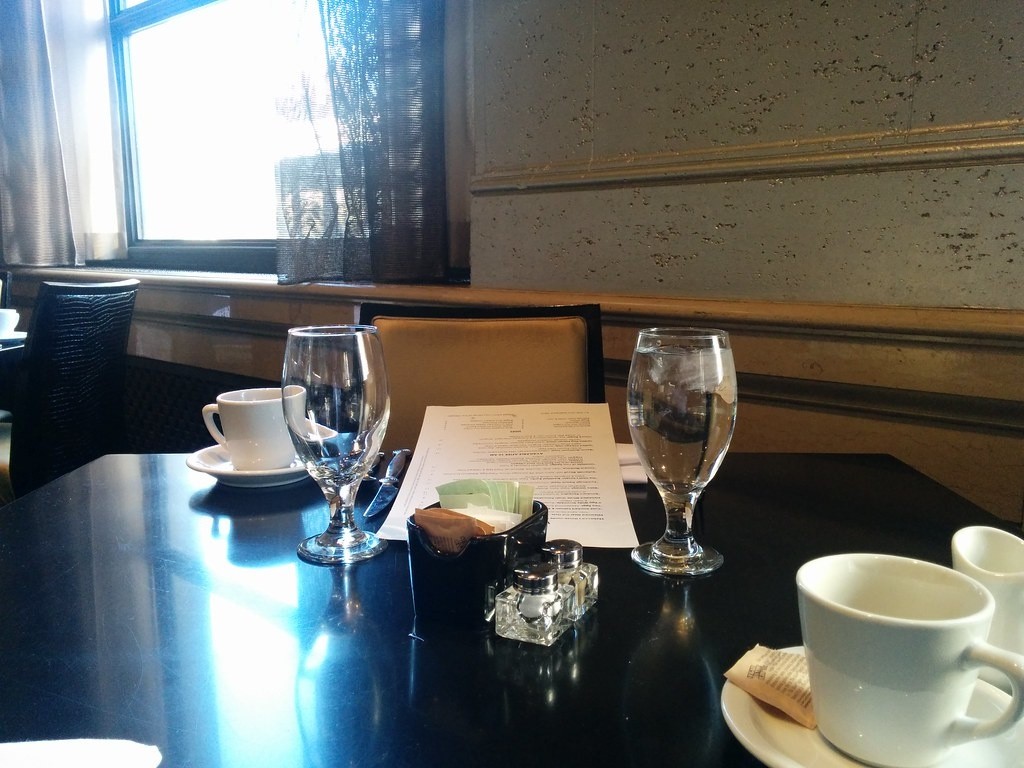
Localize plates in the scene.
[0,331,27,342]
[720,645,1024,768]
[186,444,312,487]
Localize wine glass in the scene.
[625,326,739,577]
[281,323,391,562]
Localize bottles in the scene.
[541,539,598,620]
[495,561,577,646]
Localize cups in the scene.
[202,387,295,470]
[0,309,20,335]
[794,552,1024,768]
[952,524,1024,693]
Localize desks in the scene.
[0,453,1023,768]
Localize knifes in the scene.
[363,449,411,517]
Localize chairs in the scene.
[0,275,141,504]
[353,300,610,456]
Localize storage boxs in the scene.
[405,500,548,641]
